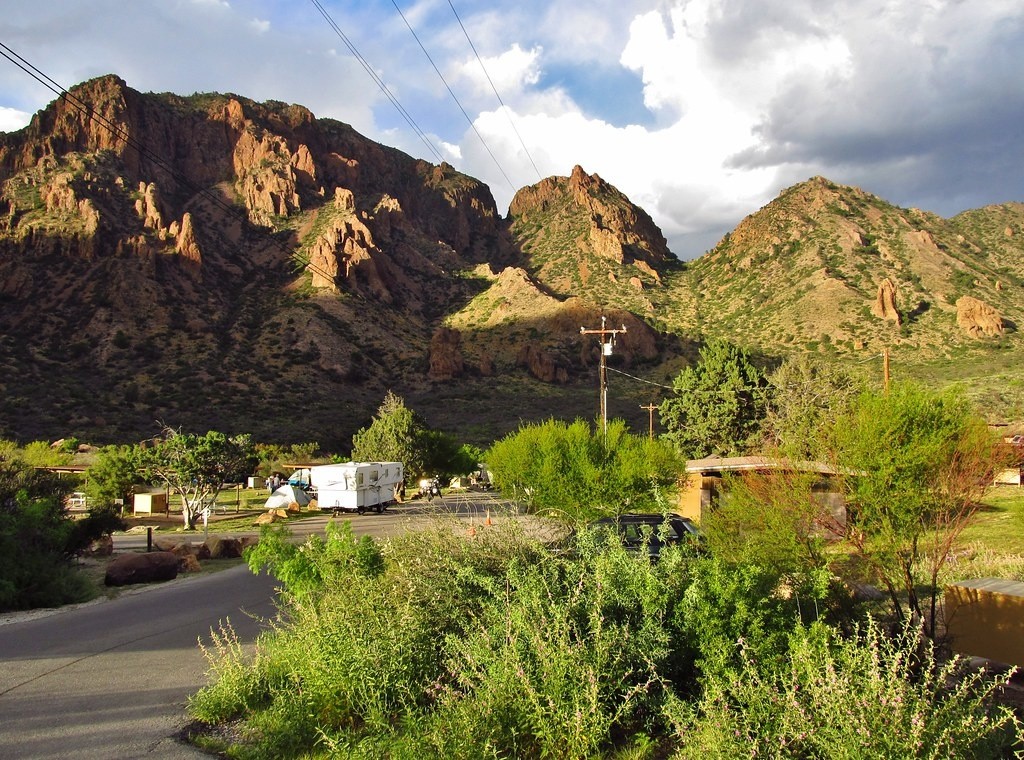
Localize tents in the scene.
[263,468,312,509]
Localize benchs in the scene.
[187,500,227,514]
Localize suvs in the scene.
[535,512,714,564]
